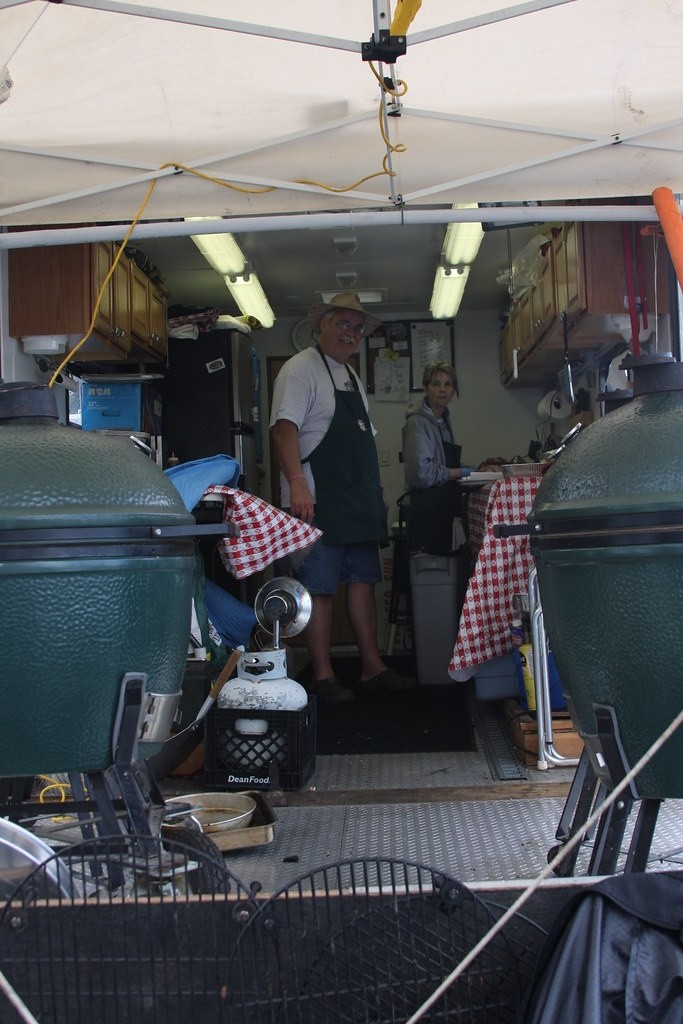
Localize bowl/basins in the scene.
[501,462,548,479]
[470,471,503,480]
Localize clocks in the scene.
[292,318,318,352]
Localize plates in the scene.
[165,793,256,834]
[457,479,494,485]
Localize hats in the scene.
[307,292,382,337]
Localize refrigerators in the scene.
[139,328,275,606]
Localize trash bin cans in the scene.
[407,541,462,686]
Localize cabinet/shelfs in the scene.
[8,222,171,376]
[499,196,670,389]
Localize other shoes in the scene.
[310,675,354,706]
[360,668,414,690]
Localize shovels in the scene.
[133,646,246,782]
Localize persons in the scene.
[267,291,416,702]
[400,364,474,555]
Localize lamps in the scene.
[183,215,277,329]
[429,203,486,320]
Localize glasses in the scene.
[325,318,366,336]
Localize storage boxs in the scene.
[207,691,316,793]
[82,382,162,437]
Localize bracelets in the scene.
[287,473,305,481]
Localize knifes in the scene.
[560,310,576,406]
